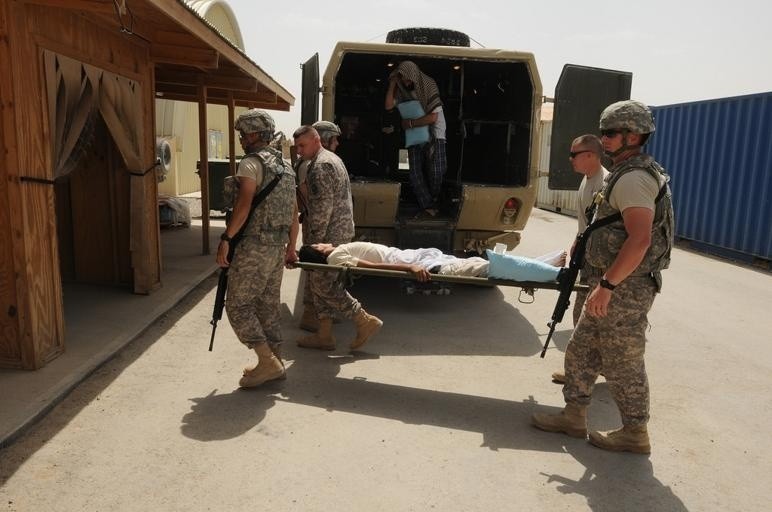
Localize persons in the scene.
[551,132,614,386]
[297,242,568,288]
[385,60,448,219]
[216,109,298,389]
[530,100,674,455]
[293,125,384,350]
[294,121,344,335]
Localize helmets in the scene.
[312,121,341,148]
[600,100,655,135]
[233,110,276,134]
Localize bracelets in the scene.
[408,118,416,128]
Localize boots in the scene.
[349,307,384,349]
[587,423,651,454]
[240,341,287,388]
[531,402,588,439]
[294,317,337,352]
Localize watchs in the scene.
[599,278,617,290]
[220,234,232,242]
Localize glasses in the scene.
[571,151,594,158]
[601,130,621,138]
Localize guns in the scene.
[209,211,245,351]
[540,228,587,359]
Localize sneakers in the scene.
[551,368,566,383]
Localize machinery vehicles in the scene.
[302,26,638,261]
